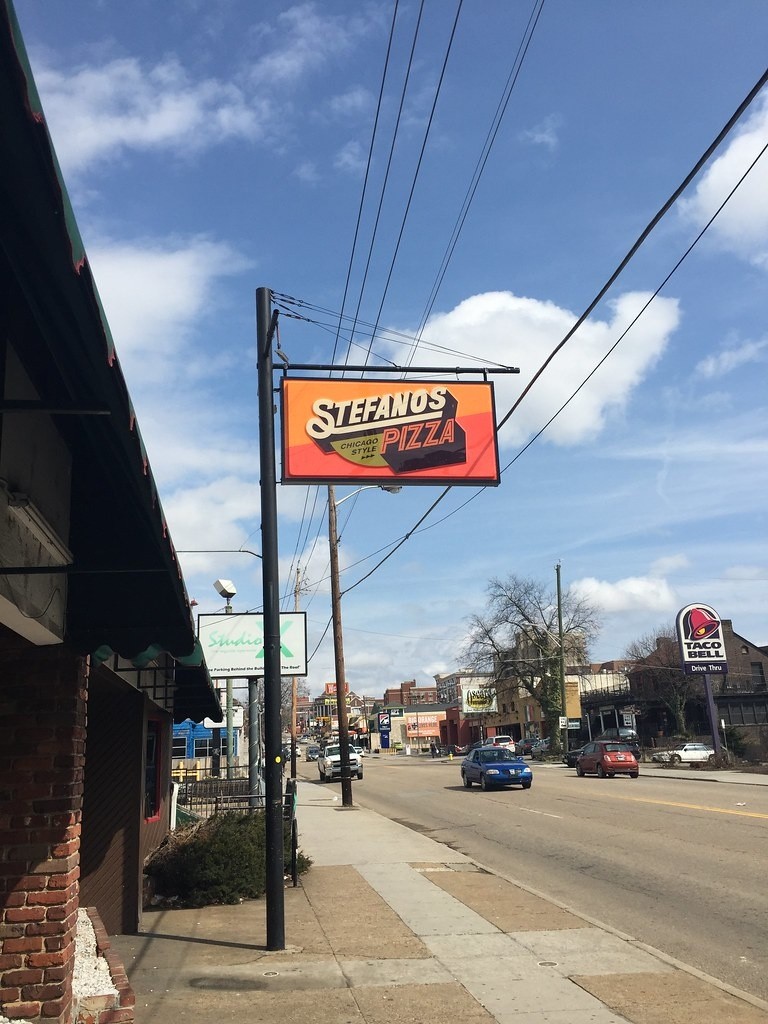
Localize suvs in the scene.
[481,735,516,754]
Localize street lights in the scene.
[328,484,405,813]
[521,623,569,757]
[213,577,238,784]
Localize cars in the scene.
[391,741,403,750]
[282,736,326,762]
[514,738,541,756]
[460,746,533,791]
[575,740,639,779]
[592,727,639,744]
[530,738,563,759]
[354,747,364,757]
[651,744,716,767]
[562,742,643,767]
[437,741,485,755]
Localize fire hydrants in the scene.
[448,753,454,761]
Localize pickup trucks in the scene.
[318,744,364,783]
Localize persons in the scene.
[430,740,438,758]
[499,752,509,760]
